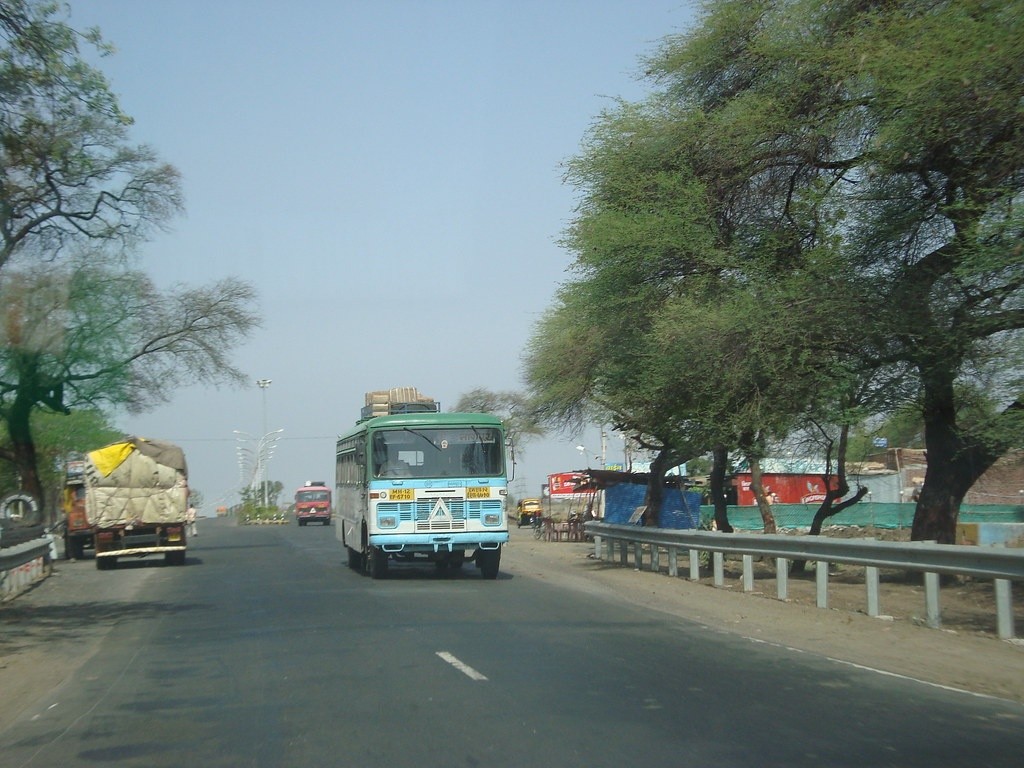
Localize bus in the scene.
[294,486,332,526]
[334,402,516,580]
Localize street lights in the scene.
[256,379,272,509]
[576,445,606,517]
[233,429,283,506]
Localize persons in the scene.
[379,446,414,478]
[186,503,199,537]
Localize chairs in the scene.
[544,519,588,542]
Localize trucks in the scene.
[517,498,541,514]
[81,437,191,570]
[61,459,95,561]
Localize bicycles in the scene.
[532,517,552,540]
